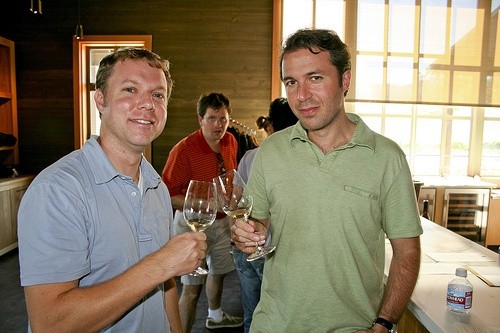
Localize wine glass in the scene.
[179,180,217,277]
[213,169,277,262]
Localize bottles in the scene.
[447,268,474,316]
[421,199,429,220]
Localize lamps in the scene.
[30,0,42,14]
[74,0,84,40]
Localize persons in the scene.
[230,99,299,333]
[161,93,244,333]
[229,30,422,333]
[18,50,207,333]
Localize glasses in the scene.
[216,153,226,175]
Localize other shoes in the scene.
[205,311,243,329]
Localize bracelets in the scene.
[371,318,398,333]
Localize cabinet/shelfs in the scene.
[0,36,18,164]
[0,178,31,258]
